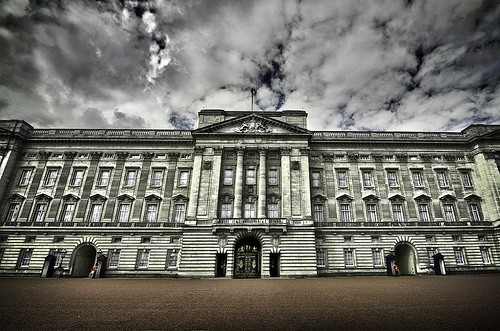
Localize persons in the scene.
[91,263,99,279]
[394,262,400,276]
